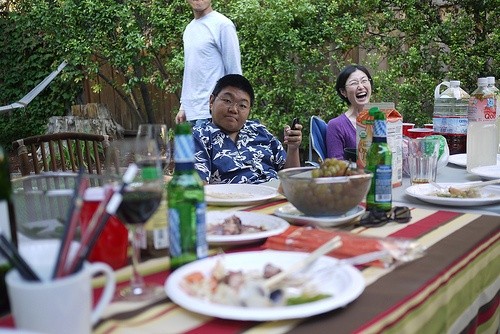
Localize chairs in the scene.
[12,132,114,228]
[307,116,329,168]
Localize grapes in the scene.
[289,157,366,216]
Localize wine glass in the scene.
[104,126,163,304]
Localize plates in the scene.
[404,181,500,206]
[206,210,291,245]
[203,185,279,206]
[165,250,366,321]
[275,203,365,227]
[449,153,500,168]
[471,165,500,180]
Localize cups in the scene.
[81,200,128,272]
[402,123,415,137]
[7,174,119,333]
[408,128,433,139]
[424,124,433,128]
[5,258,116,334]
[408,139,439,185]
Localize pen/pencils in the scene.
[0,234,44,282]
[70,163,139,273]
[48,165,85,280]
[55,177,89,277]
[62,186,115,273]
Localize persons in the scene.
[191,74,303,185]
[175,0,242,127]
[327,64,373,161]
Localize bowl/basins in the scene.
[279,166,374,217]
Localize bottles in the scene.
[133,166,169,263]
[365,107,392,213]
[433,80,470,155]
[167,124,208,273]
[0,122,17,317]
[466,78,497,174]
[486,76,500,154]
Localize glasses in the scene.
[215,95,251,112]
[355,205,413,228]
[345,77,371,88]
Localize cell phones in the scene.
[394,206,410,224]
[289,117,301,141]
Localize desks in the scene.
[0,153,500,334]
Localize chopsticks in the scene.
[460,179,500,192]
[262,236,343,289]
